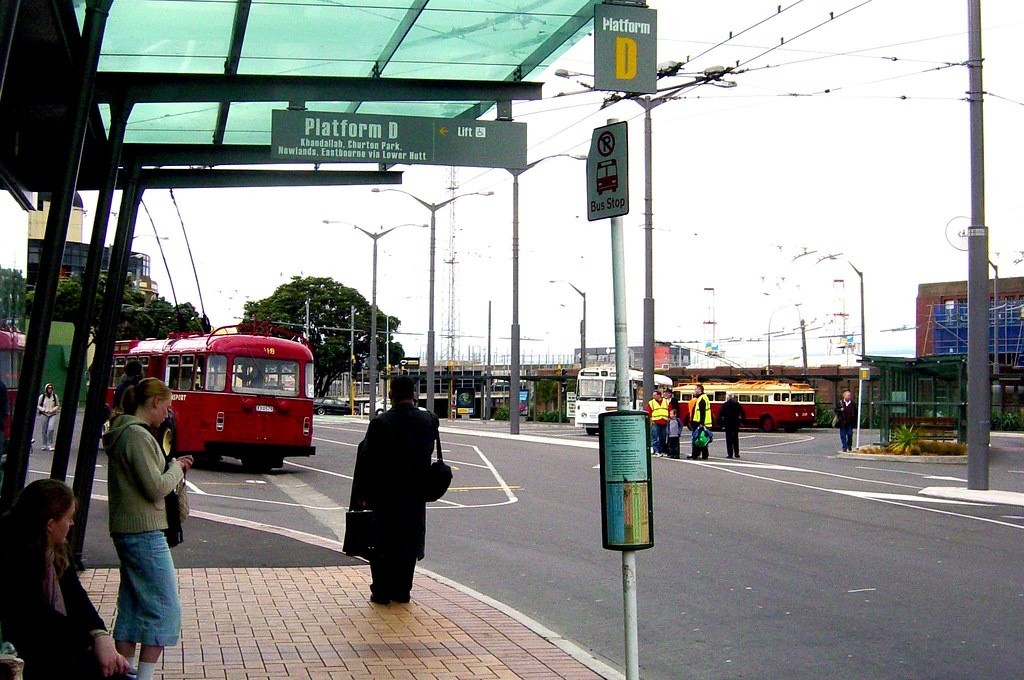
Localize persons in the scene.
[349,377,440,605]
[101,376,196,680]
[835,390,858,452]
[649,384,747,461]
[0,479,139,680]
[36,382,62,452]
[0,379,7,461]
[113,362,151,434]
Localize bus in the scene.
[574,367,674,436]
[668,379,817,434]
[98,313,316,475]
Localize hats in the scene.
[662,388,674,393]
[125,361,144,376]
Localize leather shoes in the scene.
[370,588,413,604]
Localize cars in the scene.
[310,397,359,417]
[364,397,429,418]
[340,397,371,415]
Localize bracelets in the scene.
[95,631,111,636]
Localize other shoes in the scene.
[653,448,741,461]
[41,445,55,451]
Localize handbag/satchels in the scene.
[692,424,714,447]
[343,506,383,551]
[166,492,183,546]
[830,414,843,428]
[428,458,454,503]
[174,477,190,522]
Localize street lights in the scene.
[481,150,588,438]
[323,214,433,420]
[371,185,495,419]
[846,260,874,430]
